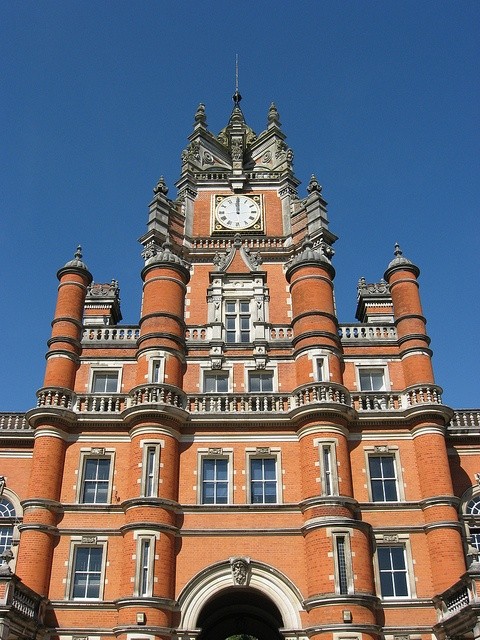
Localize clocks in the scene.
[210,193,265,237]
